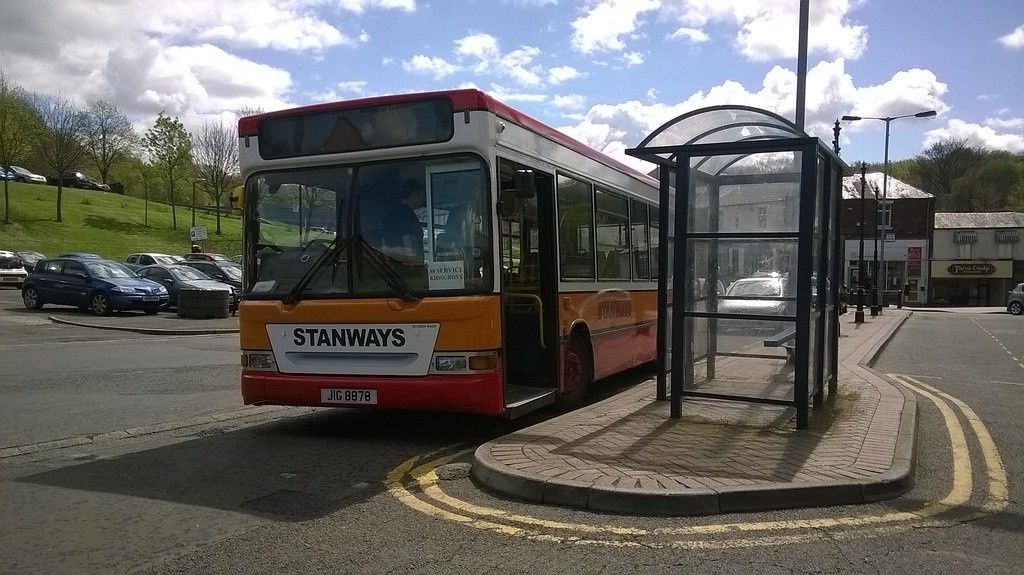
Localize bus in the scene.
[227,90,677,424]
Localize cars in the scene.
[717,277,791,336]
[173,260,243,289]
[120,253,186,273]
[1007,282,1024,315]
[23,257,171,317]
[751,268,830,309]
[0,251,29,289]
[230,254,261,268]
[16,251,49,277]
[0,166,14,181]
[183,253,242,271]
[58,252,103,260]
[135,264,239,315]
[46,172,111,191]
[3,165,47,185]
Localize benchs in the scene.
[764,326,796,347]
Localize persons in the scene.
[382,182,424,265]
[864,272,872,307]
[702,266,730,305]
[435,206,488,291]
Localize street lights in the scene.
[842,110,939,311]
[192,178,206,228]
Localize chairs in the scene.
[522,247,648,279]
